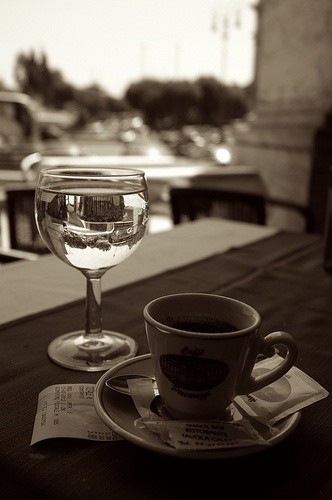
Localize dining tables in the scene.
[0,216,332,499]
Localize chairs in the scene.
[165,188,311,234]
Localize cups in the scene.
[142,293,298,421]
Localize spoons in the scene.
[103,374,161,398]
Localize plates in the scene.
[92,353,303,460]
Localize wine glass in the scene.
[34,167,149,371]
